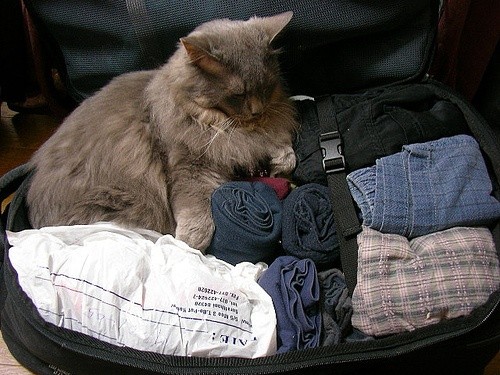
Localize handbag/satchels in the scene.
[1,0,499,375]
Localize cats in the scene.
[20,8,301,256]
